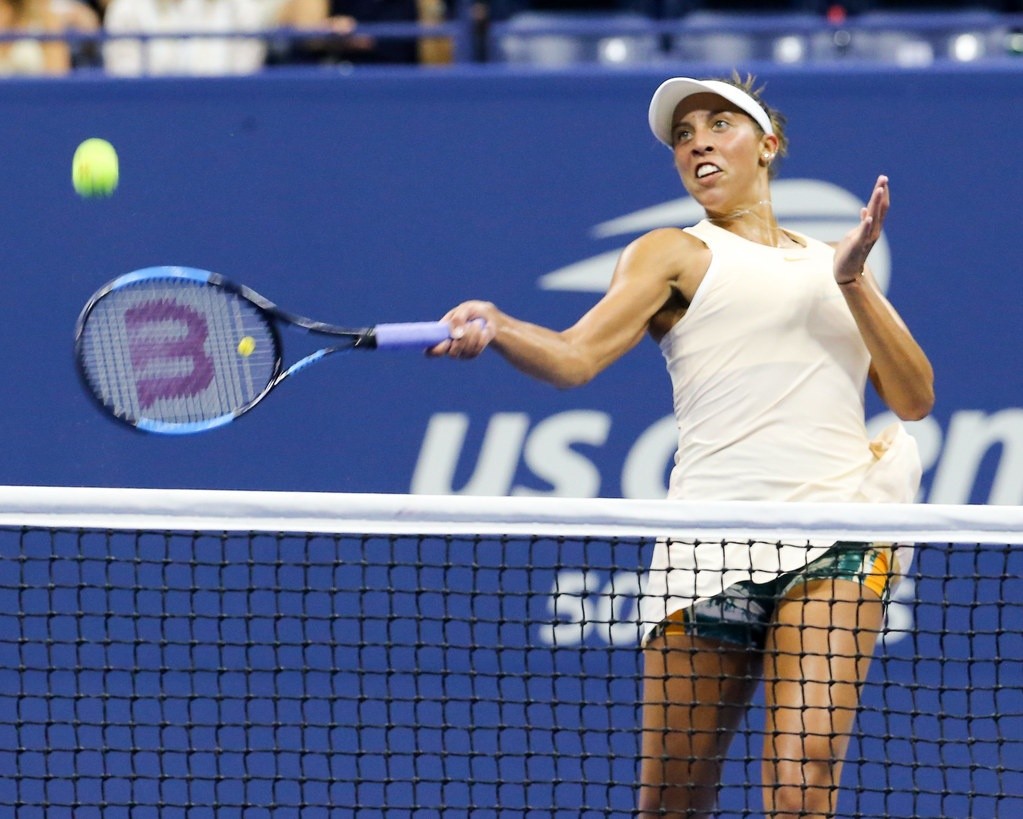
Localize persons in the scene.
[423,67,934,819]
[0,0,498,66]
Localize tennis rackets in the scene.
[71,263,487,437]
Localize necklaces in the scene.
[709,201,775,221]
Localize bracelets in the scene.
[839,265,863,288]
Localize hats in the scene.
[647,77,773,154]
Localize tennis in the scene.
[71,137,119,198]
